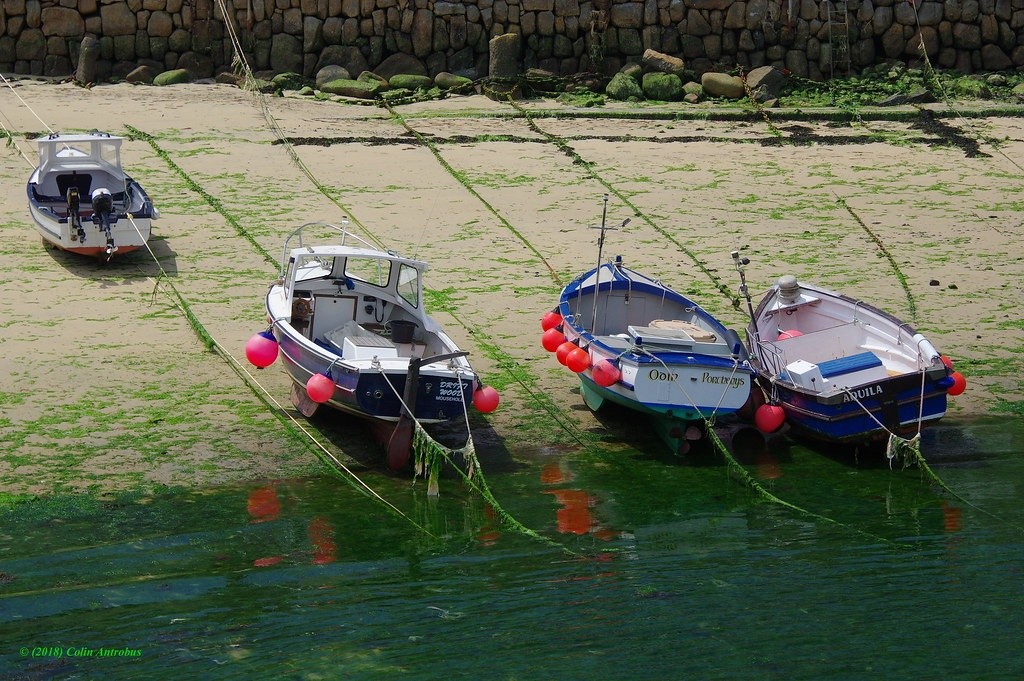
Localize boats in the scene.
[24,125,158,261]
[541,254,753,457]
[745,275,958,451]
[266,222,479,472]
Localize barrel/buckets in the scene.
[390,319,419,344]
